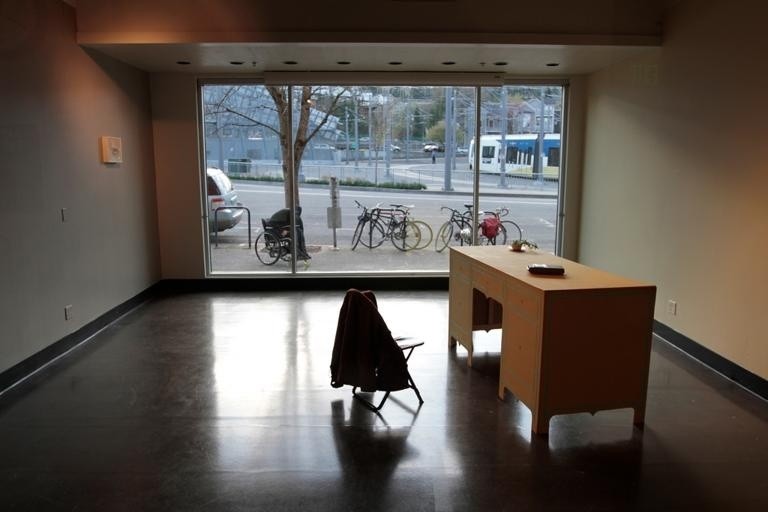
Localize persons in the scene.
[269,206,303,251]
[432,148,436,165]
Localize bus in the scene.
[467,133,561,182]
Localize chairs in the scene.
[342,288,424,411]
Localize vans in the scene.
[206,165,244,233]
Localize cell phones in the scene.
[528,263,564,275]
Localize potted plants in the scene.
[511,238,538,251]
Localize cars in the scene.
[391,142,401,152]
[423,142,439,152]
[314,141,336,152]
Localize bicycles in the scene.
[435,199,523,254]
[351,199,433,251]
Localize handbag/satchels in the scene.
[482,218,499,238]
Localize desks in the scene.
[447,244,658,435]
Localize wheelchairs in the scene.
[255,218,311,266]
